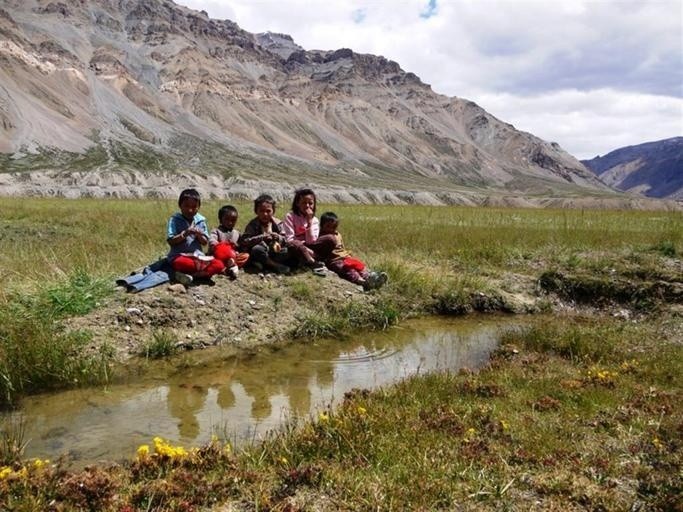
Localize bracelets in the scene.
[179,230,185,240]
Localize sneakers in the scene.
[310,261,325,268]
[252,263,289,274]
[229,258,240,278]
[175,272,194,285]
[366,271,388,290]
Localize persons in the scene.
[162,342,343,443]
[163,188,226,287]
[317,211,389,293]
[282,188,331,276]
[205,204,250,281]
[237,193,289,277]
